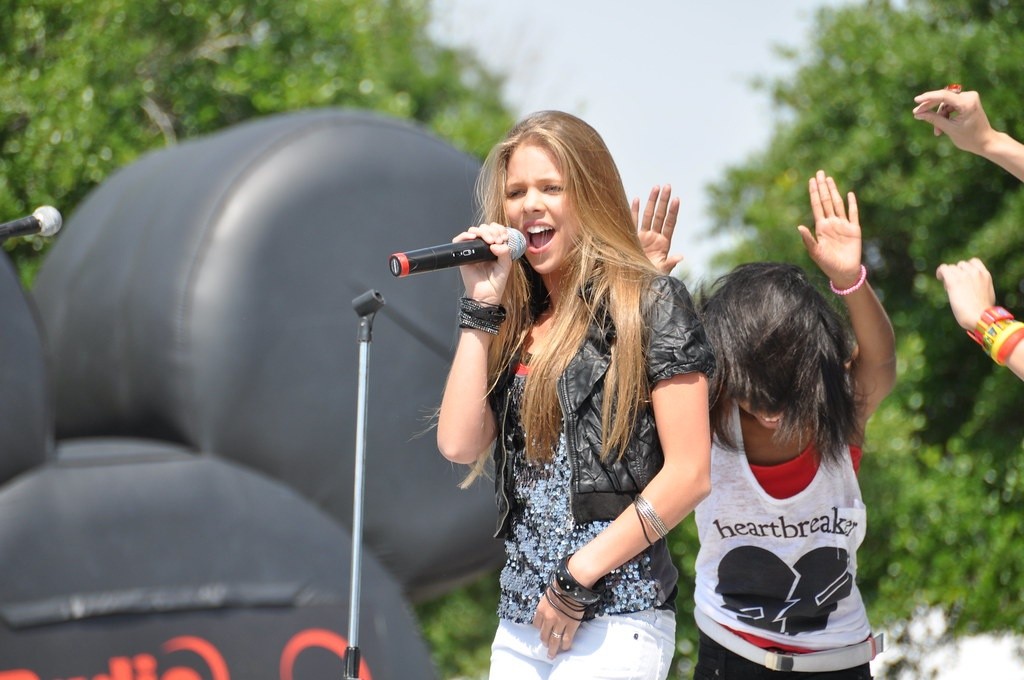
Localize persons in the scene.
[632,169,897,680]
[434,111,718,680]
[912,89,1024,381]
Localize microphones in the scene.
[389,225,527,278]
[0,205,63,240]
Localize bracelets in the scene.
[458,296,506,335]
[830,265,865,294]
[967,306,1024,368]
[632,494,669,545]
[544,553,606,622]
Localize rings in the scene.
[551,632,561,638]
[953,89,961,93]
